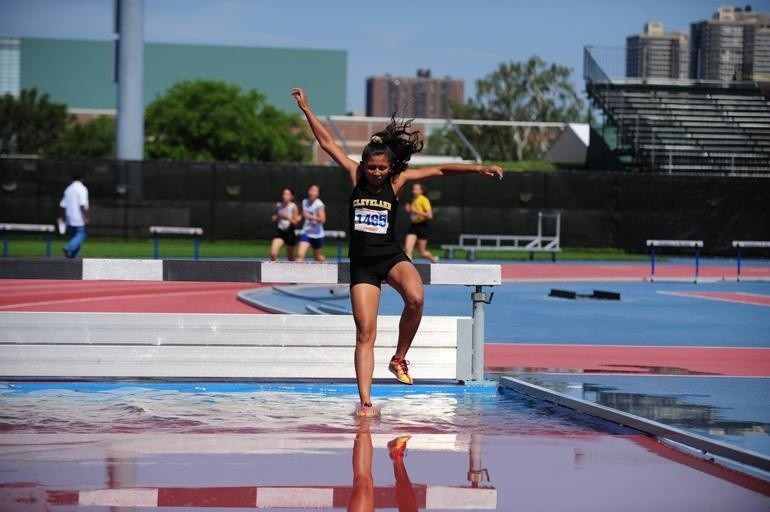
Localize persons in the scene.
[402,181,437,264]
[57,177,91,258]
[288,87,504,410]
[293,184,327,262]
[270,186,298,261]
[350,413,417,512]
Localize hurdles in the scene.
[1,257,501,381]
[0,434,497,508]
[0,222,55,259]
[149,226,204,262]
[732,240,770,275]
[646,240,704,278]
[292,230,345,262]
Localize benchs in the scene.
[583,45,768,178]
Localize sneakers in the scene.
[390,356,413,387]
[388,436,410,458]
